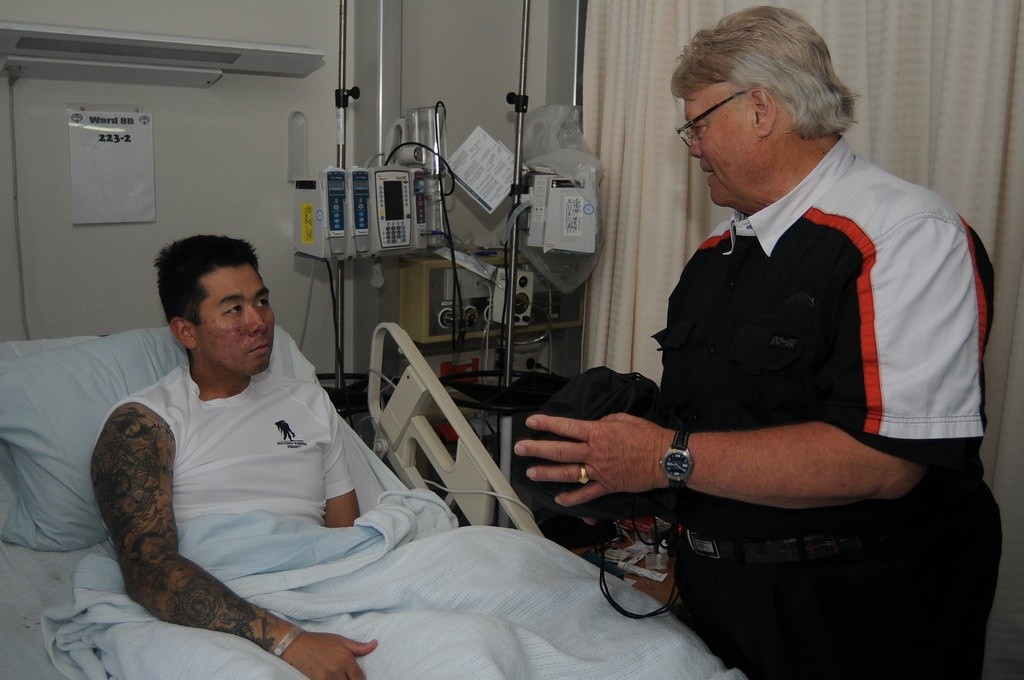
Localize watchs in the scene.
[659,430,693,490]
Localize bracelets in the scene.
[272,624,305,657]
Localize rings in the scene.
[577,463,589,485]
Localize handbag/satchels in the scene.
[529,367,663,520]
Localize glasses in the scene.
[677,87,749,146]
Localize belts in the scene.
[682,528,858,564]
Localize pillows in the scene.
[0,316,292,551]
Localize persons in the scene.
[515,4,1001,680]
[90,233,378,680]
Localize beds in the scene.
[0,322,545,680]
